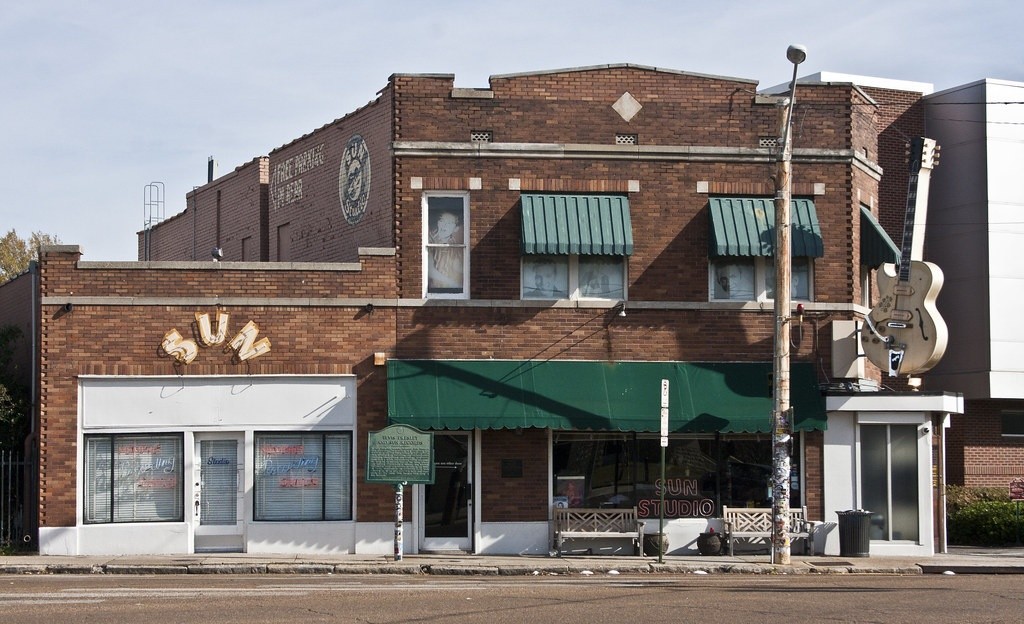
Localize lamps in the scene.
[212,247,223,262]
[614,303,626,317]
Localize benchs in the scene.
[553,506,646,557]
[723,505,816,556]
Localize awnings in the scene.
[859,207,902,270]
[707,197,824,258]
[519,192,633,256]
[387,361,829,436]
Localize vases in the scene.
[644,533,669,556]
[697,532,722,555]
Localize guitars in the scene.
[858,135,949,377]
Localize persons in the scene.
[563,481,580,508]
[524,260,568,299]
[580,258,610,298]
[766,263,802,299]
[716,261,742,297]
[428,210,462,291]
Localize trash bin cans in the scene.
[836,508,875,558]
